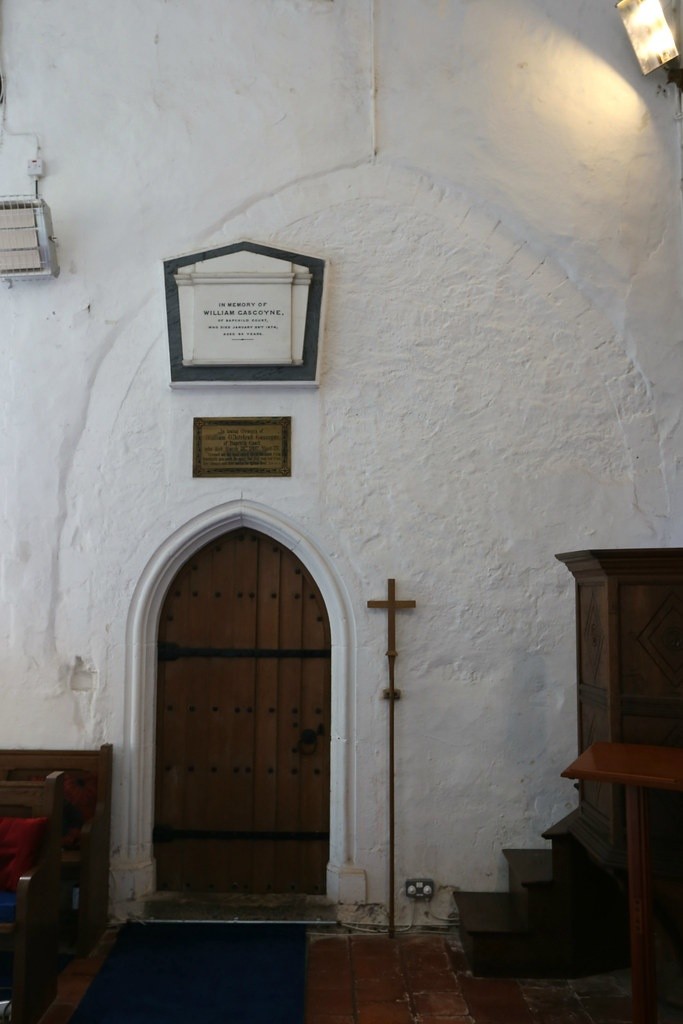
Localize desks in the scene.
[570,739,683,1024]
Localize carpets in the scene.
[65,922,308,1024]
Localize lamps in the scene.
[615,0,683,77]
[0,192,60,286]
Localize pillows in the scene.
[22,771,100,851]
[0,817,50,892]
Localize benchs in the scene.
[0,743,114,1024]
